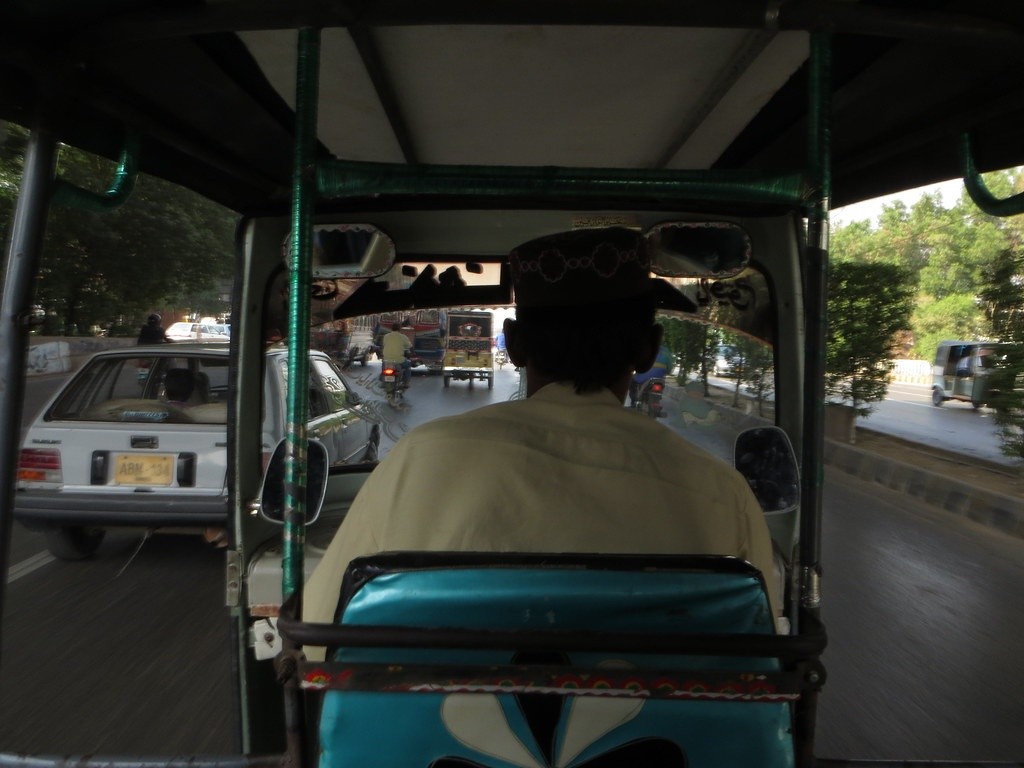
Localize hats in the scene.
[509,225,700,313]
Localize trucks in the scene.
[307,308,443,374]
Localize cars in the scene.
[164,321,231,342]
[698,342,752,378]
[10,339,386,558]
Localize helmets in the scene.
[147,313,161,322]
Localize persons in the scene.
[137,314,175,344]
[300,227,773,662]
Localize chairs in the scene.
[318,550,795,768]
[163,371,210,406]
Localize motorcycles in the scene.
[634,377,666,419]
[379,348,411,404]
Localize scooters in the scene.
[495,348,509,369]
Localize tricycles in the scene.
[930,340,1024,409]
[441,310,497,389]
